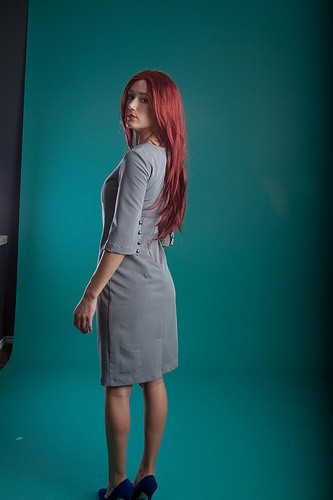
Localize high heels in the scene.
[98,478,134,500]
[134,474,158,499]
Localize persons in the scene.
[73,70,187,500]
